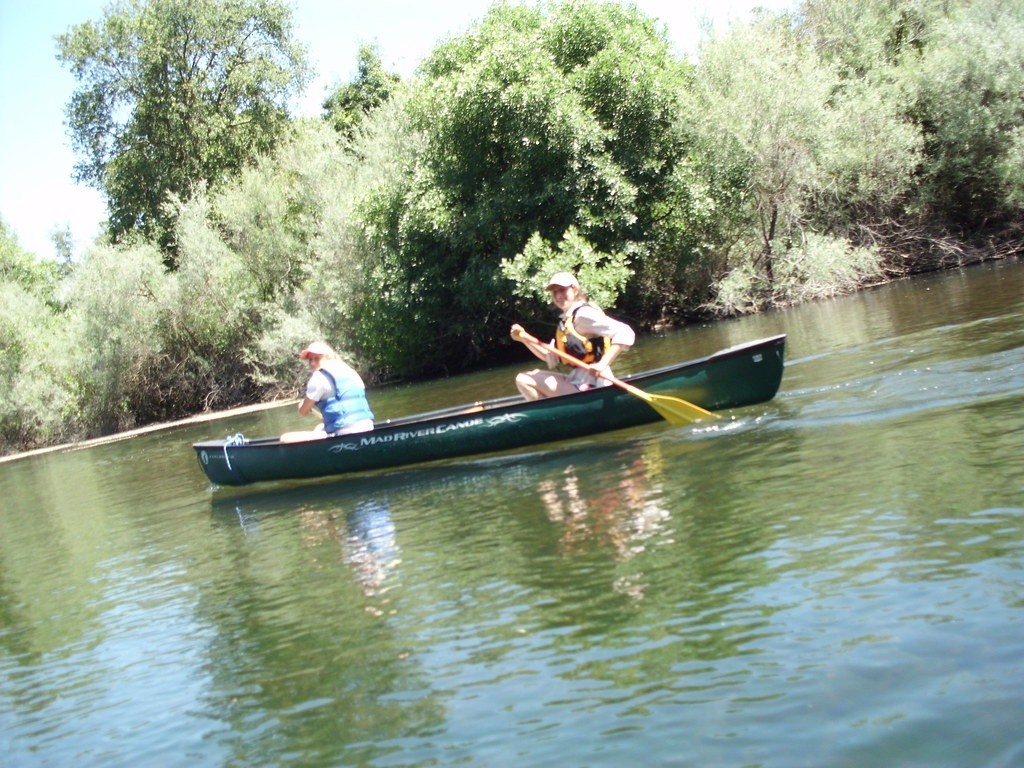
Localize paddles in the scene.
[514,327,722,427]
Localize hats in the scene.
[546,272,579,291]
[300,342,330,359]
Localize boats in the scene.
[193,331,787,483]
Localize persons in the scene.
[277,339,374,444]
[512,271,636,404]
